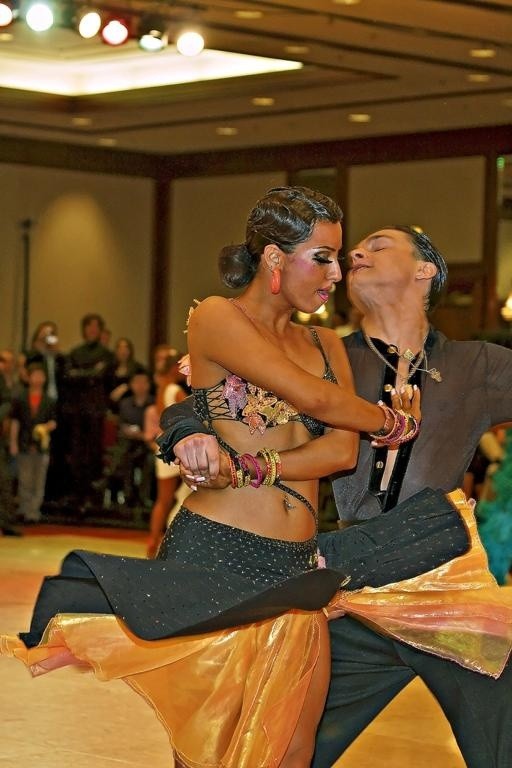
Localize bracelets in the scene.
[368,400,418,446]
[231,448,281,490]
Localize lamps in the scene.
[0,0,208,58]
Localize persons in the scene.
[155,223,511,768]
[1,313,188,557]
[1,188,422,767]
[463,430,511,588]
